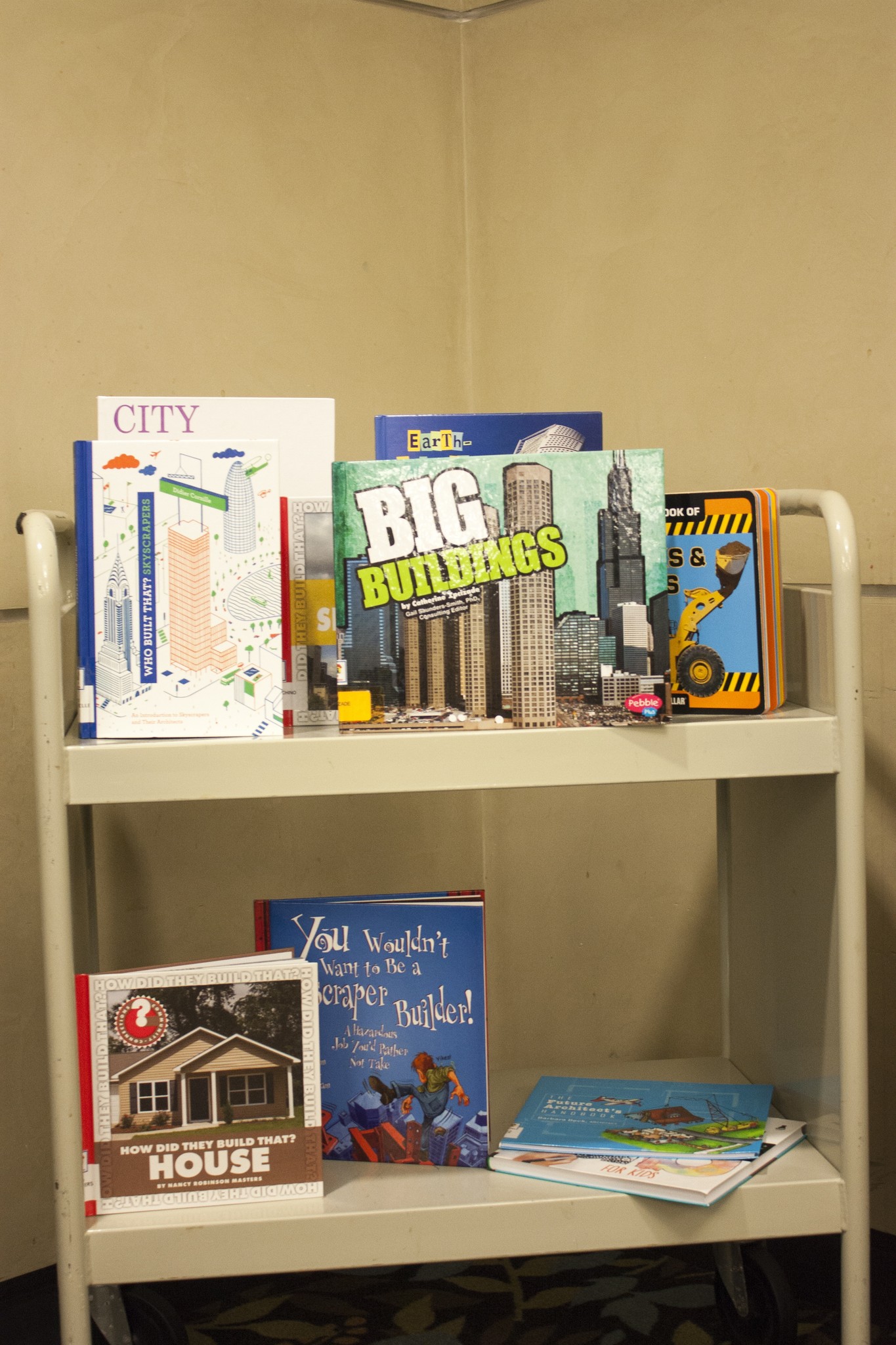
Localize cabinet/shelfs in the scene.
[15,487,869,1345]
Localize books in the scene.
[94,392,343,725]
[74,946,327,1214]
[252,887,494,1170]
[373,409,604,461]
[486,1112,810,1207]
[70,435,286,741]
[496,1073,776,1164]
[329,445,674,732]
[663,487,792,715]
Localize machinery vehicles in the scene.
[670,541,750,697]
